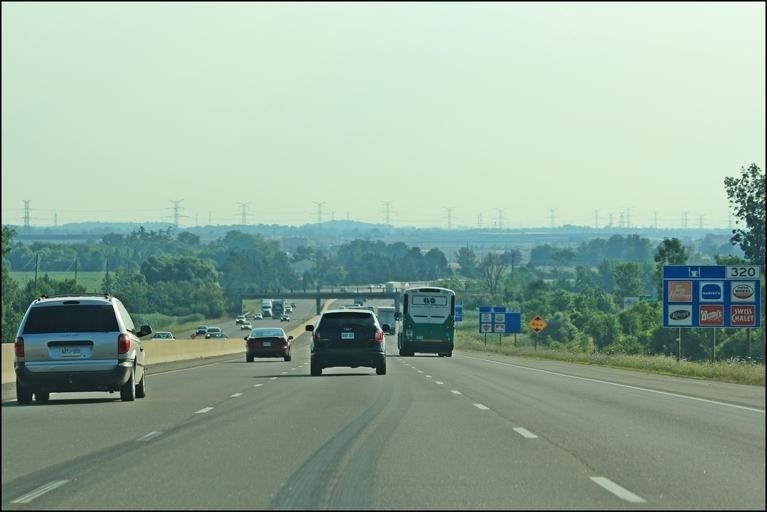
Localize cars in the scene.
[368,305,375,312]
[244,328,294,362]
[377,284,385,289]
[305,310,391,375]
[236,315,252,331]
[368,284,374,289]
[254,313,262,320]
[152,331,175,340]
[12,294,153,404]
[280,302,296,322]
[195,325,229,340]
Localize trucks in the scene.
[377,306,396,336]
[261,299,284,319]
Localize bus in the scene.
[396,287,456,357]
